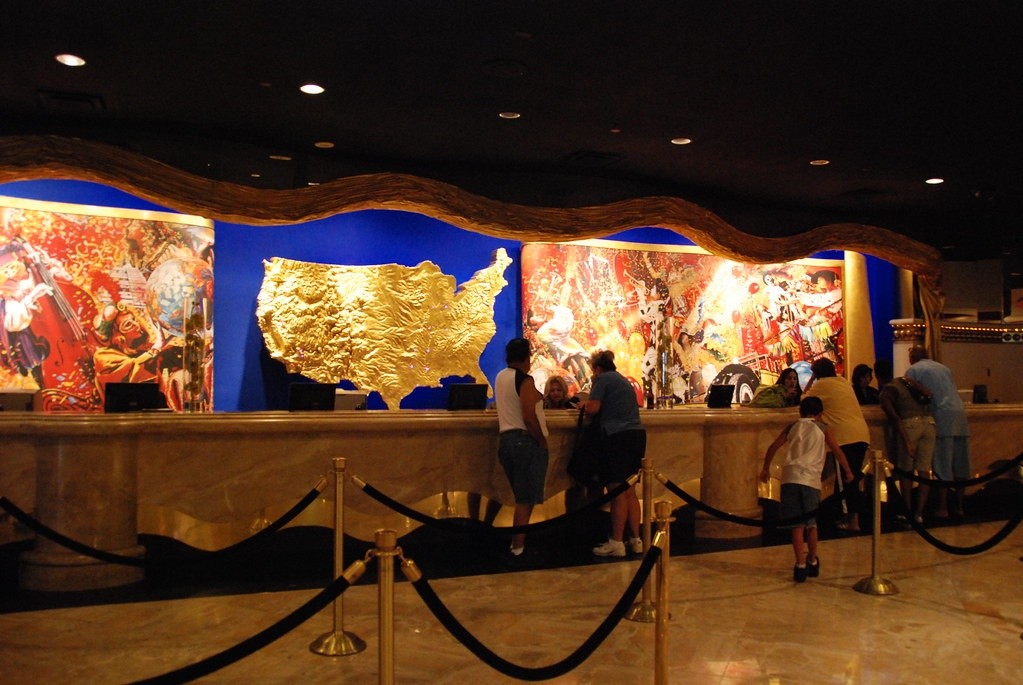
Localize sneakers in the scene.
[623,540,643,553]
[593,539,626,557]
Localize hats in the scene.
[851,364,874,385]
[504,338,532,363]
[908,344,929,359]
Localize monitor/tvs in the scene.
[288,383,336,411]
[105,382,160,414]
[973,384,988,405]
[448,383,488,411]
[707,384,736,408]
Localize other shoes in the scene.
[837,520,862,531]
[914,515,924,523]
[794,556,820,582]
[949,503,965,515]
[506,547,540,569]
[925,507,949,517]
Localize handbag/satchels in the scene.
[566,424,611,487]
[748,384,789,408]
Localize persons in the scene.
[494,339,551,565]
[775,368,802,405]
[904,345,971,518]
[467,491,503,527]
[874,357,937,523]
[851,364,881,406]
[637,278,670,404]
[543,376,572,409]
[759,396,854,582]
[578,350,647,556]
[93,303,186,413]
[800,358,871,531]
[761,267,844,355]
